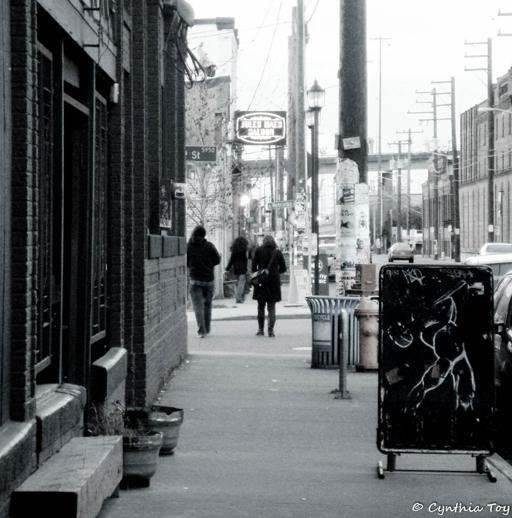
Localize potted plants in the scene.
[86,403,164,489]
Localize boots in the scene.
[268,328,275,337]
[255,328,264,335]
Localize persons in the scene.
[226,235,286,337]
[187,226,221,337]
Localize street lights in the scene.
[305,79,325,295]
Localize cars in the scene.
[456,242,512,458]
[387,241,414,264]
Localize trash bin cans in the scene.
[304,295,360,368]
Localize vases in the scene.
[125,405,184,456]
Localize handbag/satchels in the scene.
[252,271,269,291]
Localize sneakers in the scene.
[196,332,204,338]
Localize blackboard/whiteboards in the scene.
[378,263,495,455]
